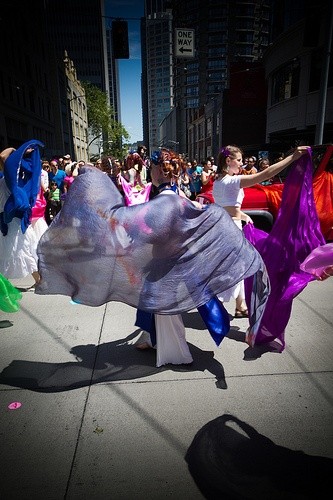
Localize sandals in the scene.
[235,308,249,318]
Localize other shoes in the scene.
[139,341,157,350]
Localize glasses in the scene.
[51,182,57,186]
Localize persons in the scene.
[123,148,234,368]
[213,144,310,317]
[0,148,286,290]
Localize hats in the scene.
[64,154,71,159]
